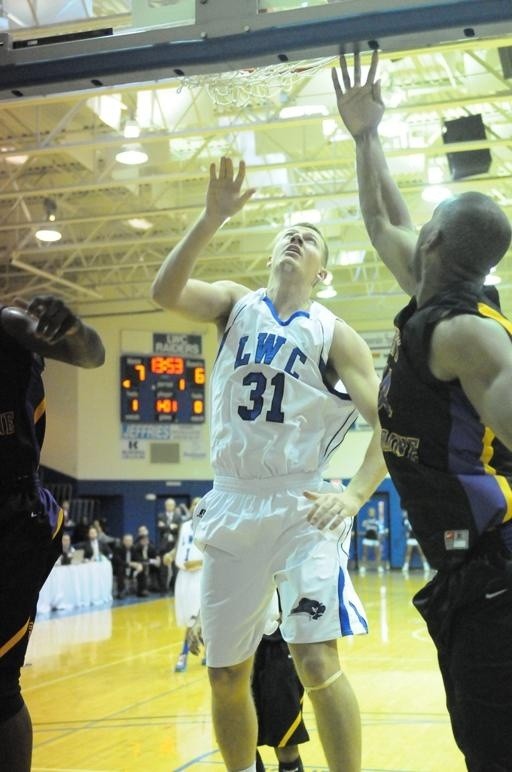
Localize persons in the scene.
[329,47,512,771]
[1,296,108,771]
[402,511,430,571]
[174,498,206,672]
[152,157,388,771]
[360,508,384,572]
[61,498,190,600]
[187,586,309,771]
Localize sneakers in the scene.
[174,652,187,672]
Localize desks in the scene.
[37,558,113,613]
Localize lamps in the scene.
[34,196,63,243]
[116,143,149,167]
